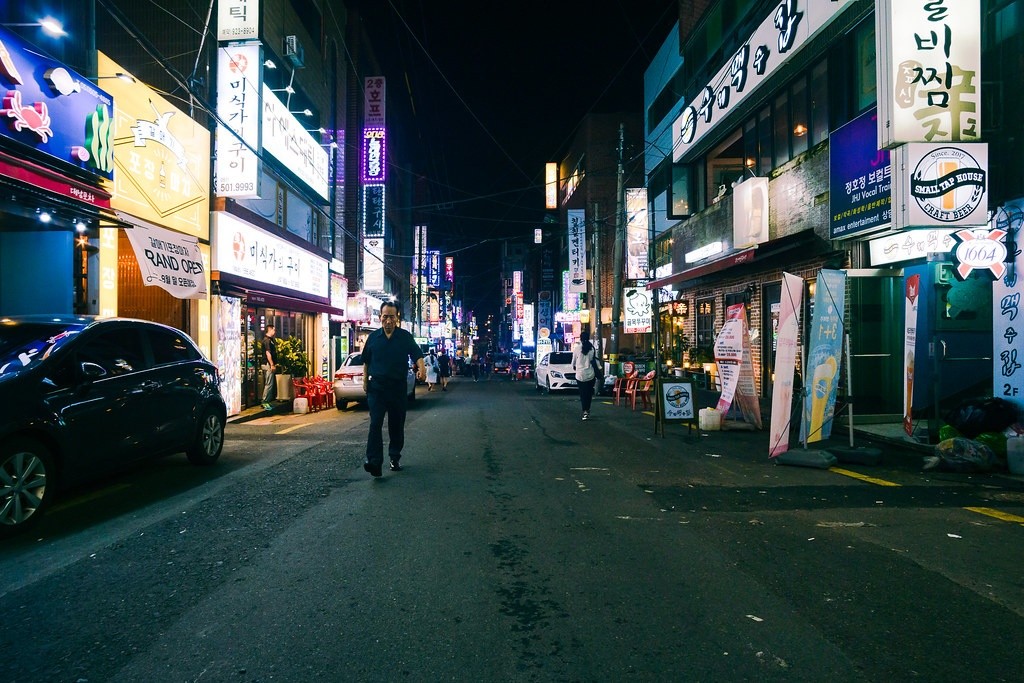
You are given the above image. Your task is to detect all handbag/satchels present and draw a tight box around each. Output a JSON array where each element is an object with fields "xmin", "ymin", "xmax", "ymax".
[
  {"xmin": 446, "ymin": 366, "xmax": 452, "ymax": 375},
  {"xmin": 433, "ymin": 364, "xmax": 440, "ymax": 374},
  {"xmin": 591, "ymin": 346, "xmax": 604, "ymax": 378}
]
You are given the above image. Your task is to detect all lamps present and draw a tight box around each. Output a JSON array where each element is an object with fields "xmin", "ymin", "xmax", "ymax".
[
  {"xmin": 1, "ymin": 16, "xmax": 66, "ymax": 34},
  {"xmin": 86, "ymin": 73, "xmax": 136, "ymax": 84},
  {"xmin": 746, "ymin": 158, "xmax": 755, "ymax": 169},
  {"xmin": 263, "ymin": 59, "xmax": 338, "ymax": 148},
  {"xmin": 793, "ymin": 125, "xmax": 807, "ymax": 136}
]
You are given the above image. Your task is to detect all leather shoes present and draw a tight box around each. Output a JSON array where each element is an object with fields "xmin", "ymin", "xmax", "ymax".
[
  {"xmin": 389, "ymin": 460, "xmax": 401, "ymax": 470},
  {"xmin": 364, "ymin": 461, "xmax": 383, "ymax": 477}
]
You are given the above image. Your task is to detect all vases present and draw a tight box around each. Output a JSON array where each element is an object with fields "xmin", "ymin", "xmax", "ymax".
[
  {"xmin": 275, "ymin": 374, "xmax": 295, "ymax": 402},
  {"xmin": 257, "ymin": 375, "xmax": 264, "ymax": 399}
]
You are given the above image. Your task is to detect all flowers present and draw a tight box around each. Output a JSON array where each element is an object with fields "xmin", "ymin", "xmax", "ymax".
[
  {"xmin": 275, "ymin": 334, "xmax": 311, "ymax": 377},
  {"xmin": 250, "ymin": 339, "xmax": 262, "ymax": 370}
]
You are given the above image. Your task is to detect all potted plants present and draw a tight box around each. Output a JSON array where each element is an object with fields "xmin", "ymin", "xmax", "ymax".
[{"xmin": 674, "ymin": 326, "xmax": 707, "ymax": 388}]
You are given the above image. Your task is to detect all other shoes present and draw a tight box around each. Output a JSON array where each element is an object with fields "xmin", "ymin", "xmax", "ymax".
[
  {"xmin": 431, "ymin": 389, "xmax": 435, "ymax": 391},
  {"xmin": 582, "ymin": 412, "xmax": 590, "ymax": 421},
  {"xmin": 428, "ymin": 385, "xmax": 431, "ymax": 391},
  {"xmin": 441, "ymin": 387, "xmax": 447, "ymax": 391}
]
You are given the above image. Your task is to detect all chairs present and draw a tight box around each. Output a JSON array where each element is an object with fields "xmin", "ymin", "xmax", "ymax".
[
  {"xmin": 613, "ymin": 370, "xmax": 656, "ymax": 411},
  {"xmin": 292, "ymin": 375, "xmax": 334, "ymax": 412}
]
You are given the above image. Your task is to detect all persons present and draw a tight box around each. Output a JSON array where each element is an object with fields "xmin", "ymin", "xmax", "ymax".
[
  {"xmin": 364, "ymin": 302, "xmax": 426, "ymax": 477},
  {"xmin": 570, "ymin": 332, "xmax": 603, "ymax": 421},
  {"xmin": 260, "ymin": 325, "xmax": 277, "ymax": 411},
  {"xmin": 424, "ymin": 347, "xmax": 519, "ymax": 391}
]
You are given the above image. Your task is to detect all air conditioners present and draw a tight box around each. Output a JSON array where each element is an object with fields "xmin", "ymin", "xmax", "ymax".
[{"xmin": 286, "ymin": 35, "xmax": 304, "ymax": 67}]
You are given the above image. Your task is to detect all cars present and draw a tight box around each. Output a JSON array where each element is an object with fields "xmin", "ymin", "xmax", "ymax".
[
  {"xmin": 0, "ymin": 313, "xmax": 227, "ymax": 537},
  {"xmin": 493, "ymin": 355, "xmax": 535, "ymax": 377},
  {"xmin": 333, "ymin": 352, "xmax": 417, "ymax": 409},
  {"xmin": 532, "ymin": 351, "xmax": 578, "ymax": 393}
]
